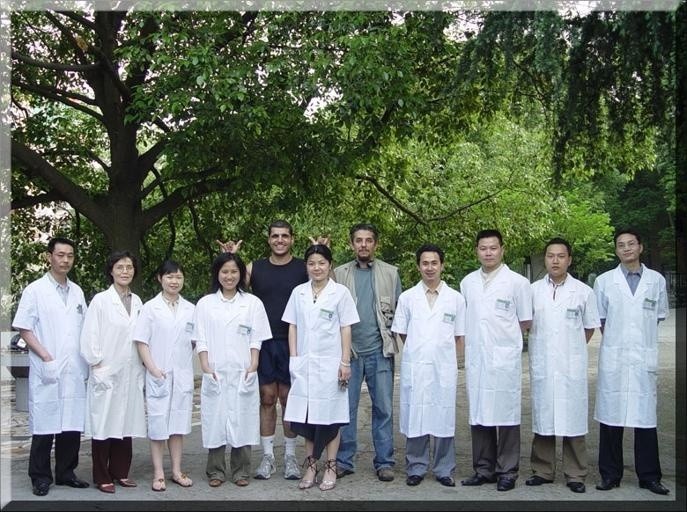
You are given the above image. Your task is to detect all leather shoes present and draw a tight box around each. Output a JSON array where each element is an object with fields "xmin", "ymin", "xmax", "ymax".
[
  {"xmin": 639, "ymin": 480, "xmax": 670, "ymax": 495},
  {"xmin": 568, "ymin": 481, "xmax": 586, "ymax": 493},
  {"xmin": 99, "ymin": 483, "xmax": 115, "ymax": 493},
  {"xmin": 32, "ymin": 480, "xmax": 49, "ymax": 496},
  {"xmin": 119, "ymin": 479, "xmax": 137, "ymax": 487},
  {"xmin": 497, "ymin": 478, "xmax": 515, "ymax": 491},
  {"xmin": 596, "ymin": 479, "xmax": 620, "ymax": 490},
  {"xmin": 461, "ymin": 473, "xmax": 494, "ymax": 485},
  {"xmin": 55, "ymin": 476, "xmax": 88, "ymax": 488},
  {"xmin": 440, "ymin": 476, "xmax": 455, "ymax": 487},
  {"xmin": 525, "ymin": 476, "xmax": 552, "ymax": 486},
  {"xmin": 406, "ymin": 476, "xmax": 421, "ymax": 486}
]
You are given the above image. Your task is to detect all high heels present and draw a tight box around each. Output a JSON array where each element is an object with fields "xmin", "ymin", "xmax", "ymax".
[
  {"xmin": 299, "ymin": 457, "xmax": 319, "ymax": 488},
  {"xmin": 319, "ymin": 459, "xmax": 338, "ymax": 490}
]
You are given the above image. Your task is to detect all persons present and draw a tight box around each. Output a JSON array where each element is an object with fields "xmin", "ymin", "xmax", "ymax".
[
  {"xmin": 593, "ymin": 229, "xmax": 673, "ymax": 494},
  {"xmin": 281, "ymin": 243, "xmax": 362, "ymax": 489},
  {"xmin": 216, "ymin": 221, "xmax": 337, "ymax": 480},
  {"xmin": 13, "ymin": 237, "xmax": 88, "ymax": 495},
  {"xmin": 134, "ymin": 261, "xmax": 195, "ymax": 491},
  {"xmin": 79, "ymin": 251, "xmax": 145, "ymax": 495},
  {"xmin": 390, "ymin": 244, "xmax": 466, "ymax": 487},
  {"xmin": 460, "ymin": 230, "xmax": 532, "ymax": 492},
  {"xmin": 331, "ymin": 225, "xmax": 402, "ymax": 481},
  {"xmin": 193, "ymin": 252, "xmax": 274, "ymax": 486},
  {"xmin": 526, "ymin": 238, "xmax": 601, "ymax": 493}
]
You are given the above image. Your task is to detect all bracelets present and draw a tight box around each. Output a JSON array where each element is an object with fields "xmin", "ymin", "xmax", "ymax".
[{"xmin": 341, "ymin": 360, "xmax": 351, "ymax": 367}]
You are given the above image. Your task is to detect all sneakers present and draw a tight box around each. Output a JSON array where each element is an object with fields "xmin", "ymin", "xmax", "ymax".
[
  {"xmin": 334, "ymin": 464, "xmax": 353, "ymax": 478},
  {"xmin": 254, "ymin": 453, "xmax": 278, "ymax": 478},
  {"xmin": 238, "ymin": 478, "xmax": 249, "ymax": 487},
  {"xmin": 377, "ymin": 466, "xmax": 393, "ymax": 481},
  {"xmin": 210, "ymin": 479, "xmax": 222, "ymax": 487},
  {"xmin": 283, "ymin": 455, "xmax": 301, "ymax": 479}
]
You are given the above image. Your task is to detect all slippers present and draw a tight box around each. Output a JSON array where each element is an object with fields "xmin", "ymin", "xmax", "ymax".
[
  {"xmin": 152, "ymin": 478, "xmax": 167, "ymax": 492},
  {"xmin": 176, "ymin": 473, "xmax": 192, "ymax": 487}
]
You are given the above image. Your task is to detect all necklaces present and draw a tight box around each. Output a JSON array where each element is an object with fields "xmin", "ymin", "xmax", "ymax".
[
  {"xmin": 313, "ymin": 286, "xmax": 323, "ymax": 305},
  {"xmin": 163, "ymin": 294, "xmax": 181, "ymax": 306}
]
